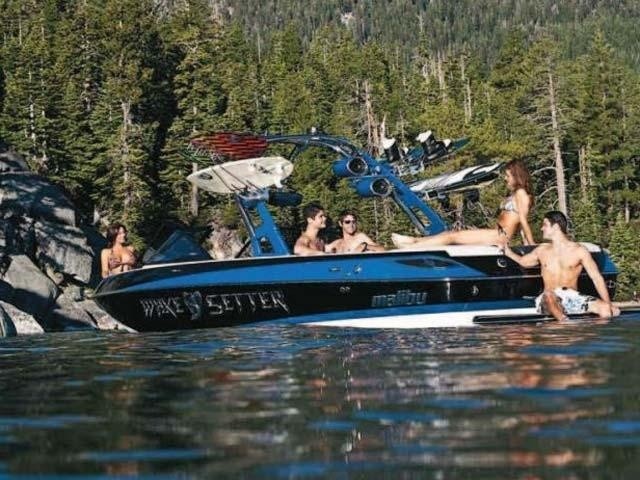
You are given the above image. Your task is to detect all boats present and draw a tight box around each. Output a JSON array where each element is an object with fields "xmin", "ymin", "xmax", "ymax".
[{"xmin": 92, "ymin": 125, "xmax": 621, "ymax": 332}]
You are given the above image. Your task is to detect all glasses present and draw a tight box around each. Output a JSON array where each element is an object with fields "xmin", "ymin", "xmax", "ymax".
[{"xmin": 343, "ymin": 220, "xmax": 356, "ymax": 224}]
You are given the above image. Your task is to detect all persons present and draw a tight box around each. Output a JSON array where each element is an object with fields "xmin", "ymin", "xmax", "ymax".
[
  {"xmin": 293, "ymin": 202, "xmax": 384, "ymax": 256},
  {"xmin": 499, "ymin": 211, "xmax": 621, "ymax": 322},
  {"xmin": 391, "ymin": 158, "xmax": 539, "ymax": 249},
  {"xmin": 100, "ymin": 224, "xmax": 136, "ymax": 279}
]
